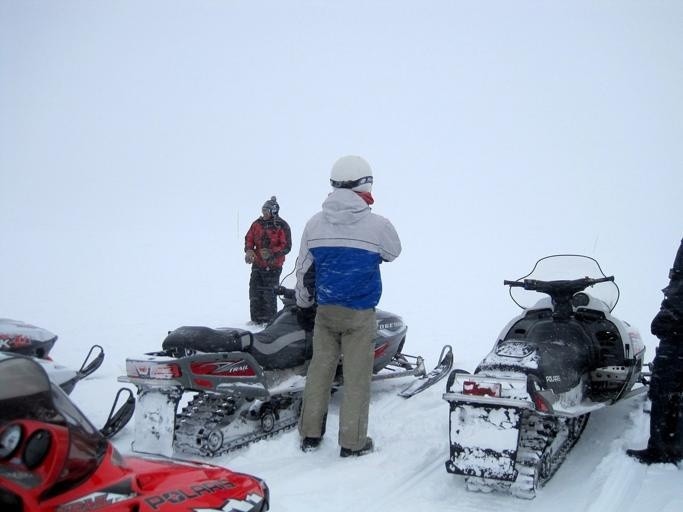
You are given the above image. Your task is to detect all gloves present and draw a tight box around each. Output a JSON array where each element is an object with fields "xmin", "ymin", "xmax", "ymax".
[
  {"xmin": 650, "ymin": 300, "xmax": 677, "ymax": 341},
  {"xmin": 245, "ymin": 250, "xmax": 257, "ymax": 264},
  {"xmin": 260, "ymin": 248, "xmax": 273, "ymax": 262},
  {"xmin": 296, "ymin": 302, "xmax": 318, "ymax": 332}
]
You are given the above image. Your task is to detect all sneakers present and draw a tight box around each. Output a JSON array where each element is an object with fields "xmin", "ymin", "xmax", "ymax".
[
  {"xmin": 626, "ymin": 446, "xmax": 680, "ymax": 466},
  {"xmin": 340, "ymin": 438, "xmax": 372, "ymax": 458},
  {"xmin": 301, "ymin": 436, "xmax": 321, "ymax": 452}
]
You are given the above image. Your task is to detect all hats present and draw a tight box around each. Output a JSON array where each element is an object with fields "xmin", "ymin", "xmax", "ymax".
[{"xmin": 262, "ymin": 197, "xmax": 279, "ymax": 217}]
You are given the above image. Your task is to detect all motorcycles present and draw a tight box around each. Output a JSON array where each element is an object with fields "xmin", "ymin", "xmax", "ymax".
[
  {"xmin": 440, "ymin": 253, "xmax": 652, "ymax": 502},
  {"xmin": 1, "ymin": 356, "xmax": 270, "ymax": 512},
  {"xmin": 0, "ymin": 317, "xmax": 136, "ymax": 441},
  {"xmin": 117, "ymin": 285, "xmax": 452, "ymax": 461}
]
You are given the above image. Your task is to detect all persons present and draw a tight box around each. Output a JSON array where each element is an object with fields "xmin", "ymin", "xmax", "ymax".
[
  {"xmin": 626, "ymin": 237, "xmax": 683, "ymax": 464},
  {"xmin": 294, "ymin": 153, "xmax": 404, "ymax": 457},
  {"xmin": 244, "ymin": 195, "xmax": 293, "ymax": 327}
]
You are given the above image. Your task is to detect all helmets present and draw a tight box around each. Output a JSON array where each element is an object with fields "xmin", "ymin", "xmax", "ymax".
[{"xmin": 330, "ymin": 155, "xmax": 374, "ymax": 193}]
